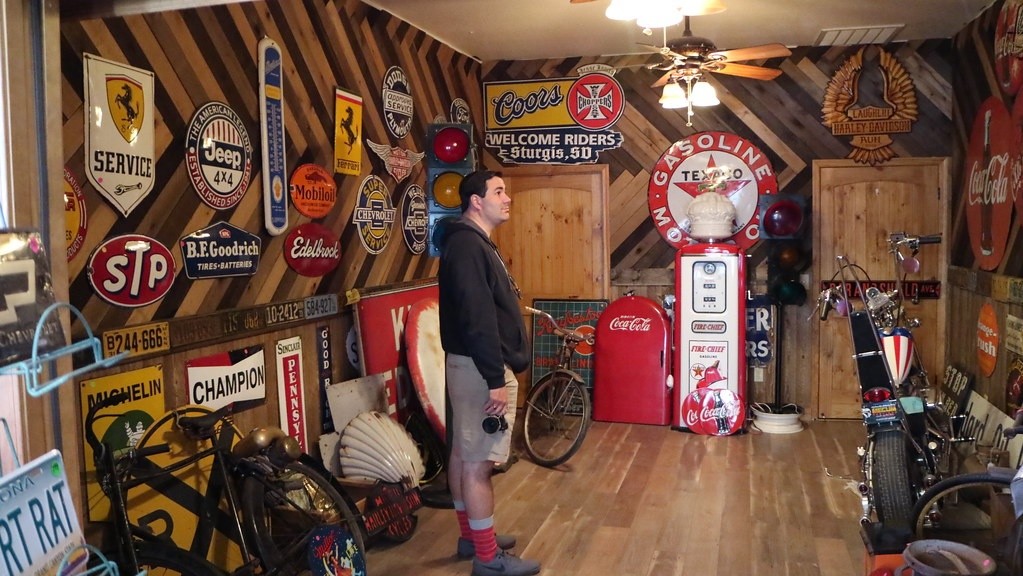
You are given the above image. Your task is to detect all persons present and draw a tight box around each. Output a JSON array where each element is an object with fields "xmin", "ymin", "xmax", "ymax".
[{"xmin": 431, "ymin": 171, "xmax": 541, "ymax": 576}]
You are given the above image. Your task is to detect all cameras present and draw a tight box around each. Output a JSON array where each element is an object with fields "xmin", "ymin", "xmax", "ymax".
[{"xmin": 481, "ymin": 406, "xmax": 508, "ymax": 434}]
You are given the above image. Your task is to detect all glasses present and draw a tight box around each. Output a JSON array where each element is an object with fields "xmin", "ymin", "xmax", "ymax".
[{"xmin": 509, "ymin": 274, "xmax": 522, "ymax": 299}]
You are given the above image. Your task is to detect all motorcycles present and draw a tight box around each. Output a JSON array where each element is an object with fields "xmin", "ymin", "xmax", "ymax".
[{"xmin": 807, "ymin": 232, "xmax": 978, "ymax": 543}]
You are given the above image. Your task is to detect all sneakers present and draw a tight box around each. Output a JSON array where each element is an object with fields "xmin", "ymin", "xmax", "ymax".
[
  {"xmin": 457, "ymin": 532, "xmax": 516, "ymax": 560},
  {"xmin": 471, "ymin": 546, "xmax": 541, "ymax": 576}
]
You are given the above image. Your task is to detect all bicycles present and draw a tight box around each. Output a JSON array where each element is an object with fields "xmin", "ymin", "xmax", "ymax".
[
  {"xmin": 521, "ymin": 306, "xmax": 596, "ymax": 468},
  {"xmin": 909, "ymin": 401, "xmax": 1023, "ymax": 576},
  {"xmin": 83, "ymin": 393, "xmax": 367, "ymax": 576}
]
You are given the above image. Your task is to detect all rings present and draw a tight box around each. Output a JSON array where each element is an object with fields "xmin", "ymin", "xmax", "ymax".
[{"xmin": 493, "ymin": 405, "xmax": 497, "ymax": 409}]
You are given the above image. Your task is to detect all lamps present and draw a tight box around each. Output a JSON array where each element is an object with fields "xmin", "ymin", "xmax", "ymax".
[
  {"xmin": 605, "ymin": 0, "xmax": 727, "ymax": 28},
  {"xmin": 658, "ymin": 72, "xmax": 720, "ymax": 109}
]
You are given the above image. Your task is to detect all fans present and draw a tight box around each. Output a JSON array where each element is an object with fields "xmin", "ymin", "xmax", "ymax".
[{"xmin": 568, "ymin": 0, "xmax": 793, "ymax": 88}]
